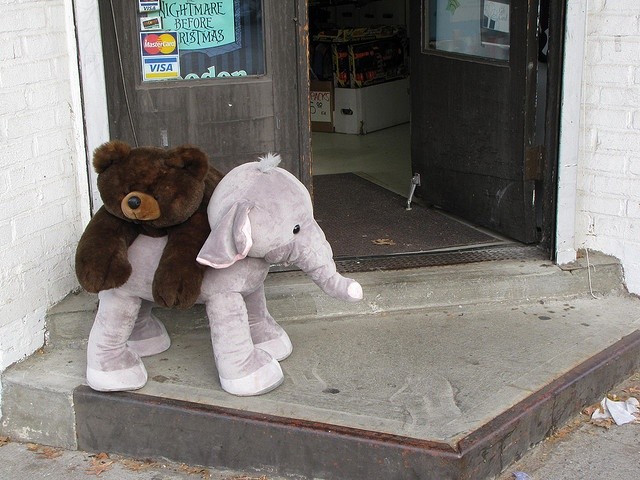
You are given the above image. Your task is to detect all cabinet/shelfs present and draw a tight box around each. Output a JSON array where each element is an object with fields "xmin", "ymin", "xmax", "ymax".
[{"xmin": 311, "ymin": 37, "xmax": 409, "ymax": 135}]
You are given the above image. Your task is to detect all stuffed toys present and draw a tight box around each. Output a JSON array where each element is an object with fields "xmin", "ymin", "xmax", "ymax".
[
  {"xmin": 86, "ymin": 153, "xmax": 363, "ymax": 394},
  {"xmin": 76, "ymin": 142, "xmax": 223, "ymax": 307}
]
